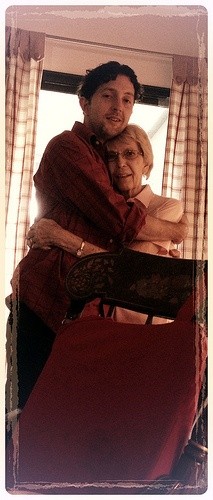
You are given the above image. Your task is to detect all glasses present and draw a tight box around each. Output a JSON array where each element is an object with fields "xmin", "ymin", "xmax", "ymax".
[{"xmin": 108, "ymin": 150, "xmax": 143, "ymax": 162}]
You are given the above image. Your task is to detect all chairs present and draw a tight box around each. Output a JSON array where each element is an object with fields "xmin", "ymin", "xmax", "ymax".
[{"xmin": 15, "ymin": 248, "xmax": 208, "ymax": 492}]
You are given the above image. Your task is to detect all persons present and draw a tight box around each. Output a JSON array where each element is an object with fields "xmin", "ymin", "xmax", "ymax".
[
  {"xmin": 26, "ymin": 123, "xmax": 184, "ymax": 325},
  {"xmin": 2, "ymin": 61, "xmax": 190, "ymax": 439}
]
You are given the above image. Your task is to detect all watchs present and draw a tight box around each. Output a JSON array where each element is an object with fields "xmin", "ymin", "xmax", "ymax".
[{"xmin": 73, "ymin": 239, "xmax": 87, "ymax": 259}]
